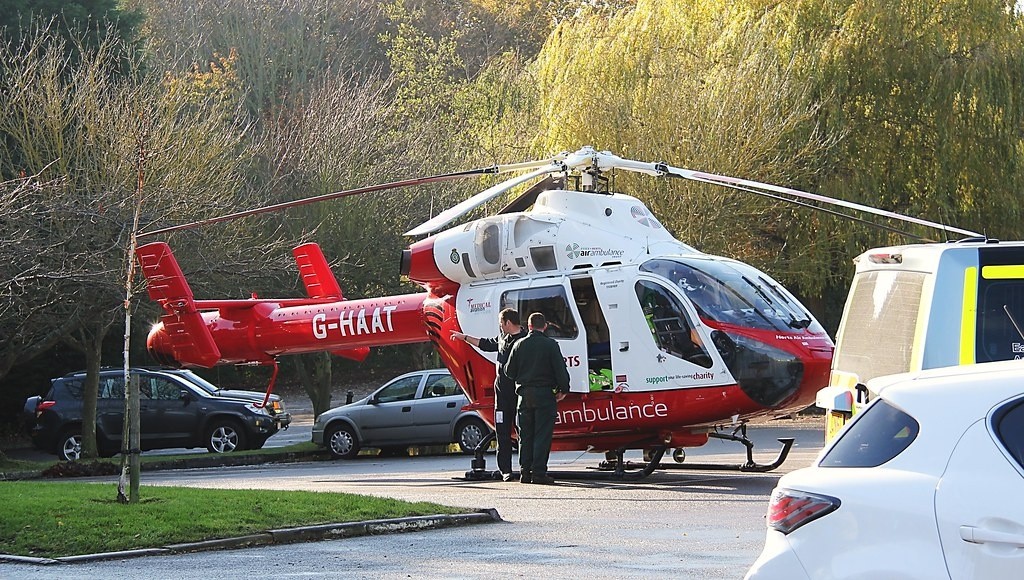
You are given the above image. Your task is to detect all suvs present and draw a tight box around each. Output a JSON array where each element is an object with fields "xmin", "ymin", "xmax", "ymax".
[
  {"xmin": 24, "ymin": 366, "xmax": 294, "ymax": 465},
  {"xmin": 745, "ymin": 357, "xmax": 1024, "ymax": 580}
]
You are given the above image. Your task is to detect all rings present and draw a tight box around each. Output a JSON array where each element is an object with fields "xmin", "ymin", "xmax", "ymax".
[{"xmin": 452, "ymin": 333, "xmax": 454, "ymax": 338}]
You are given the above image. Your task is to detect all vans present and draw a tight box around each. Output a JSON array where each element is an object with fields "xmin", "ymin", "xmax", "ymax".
[{"xmin": 815, "ymin": 239, "xmax": 1024, "ymax": 454}]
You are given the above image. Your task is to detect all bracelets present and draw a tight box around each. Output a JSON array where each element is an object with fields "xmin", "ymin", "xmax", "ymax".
[{"xmin": 464, "ymin": 334, "xmax": 469, "ymax": 341}]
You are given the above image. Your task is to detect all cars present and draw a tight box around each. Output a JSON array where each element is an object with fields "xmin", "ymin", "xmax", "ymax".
[{"xmin": 311, "ymin": 366, "xmax": 494, "ymax": 460}]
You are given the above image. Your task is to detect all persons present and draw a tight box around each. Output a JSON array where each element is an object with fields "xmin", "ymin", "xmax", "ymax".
[
  {"xmin": 503, "ymin": 313, "xmax": 570, "ymax": 484},
  {"xmin": 450, "ymin": 308, "xmax": 528, "ymax": 482},
  {"xmin": 643, "ymin": 280, "xmax": 728, "ymax": 350}
]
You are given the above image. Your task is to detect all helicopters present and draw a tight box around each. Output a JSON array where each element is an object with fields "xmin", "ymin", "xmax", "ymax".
[{"xmin": 133, "ymin": 144, "xmax": 985, "ymax": 481}]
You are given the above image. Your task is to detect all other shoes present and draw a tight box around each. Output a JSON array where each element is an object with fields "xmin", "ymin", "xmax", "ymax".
[
  {"xmin": 502, "ymin": 472, "xmax": 514, "ymax": 481},
  {"xmin": 532, "ymin": 474, "xmax": 554, "ymax": 485},
  {"xmin": 520, "ymin": 472, "xmax": 535, "ymax": 483}
]
